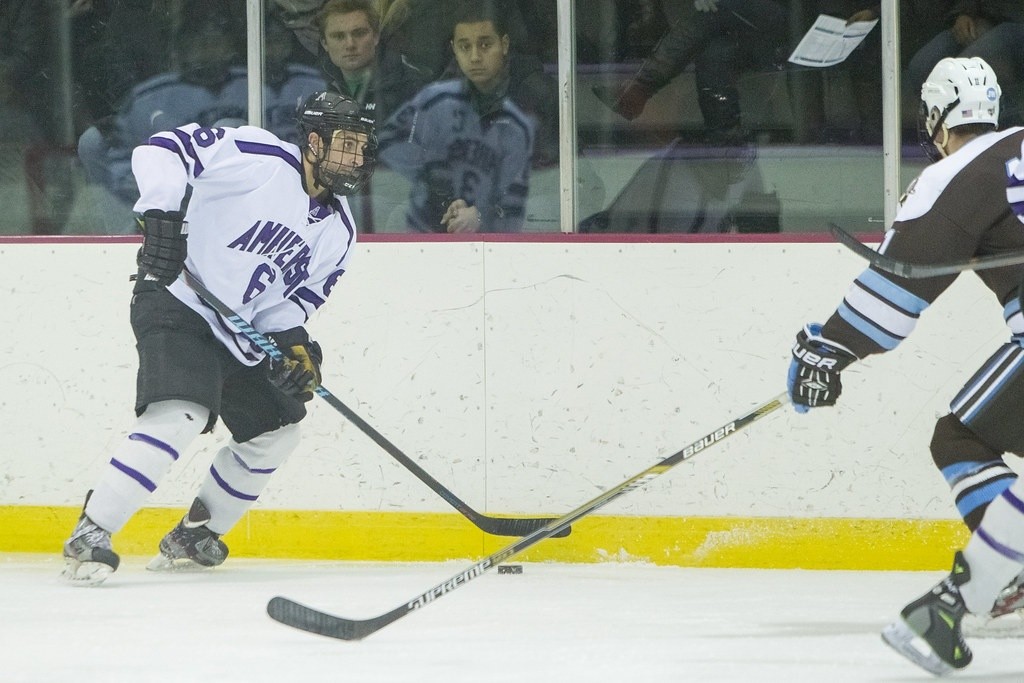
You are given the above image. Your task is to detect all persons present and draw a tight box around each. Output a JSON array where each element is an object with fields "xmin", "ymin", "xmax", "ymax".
[
  {"xmin": 787, "ymin": 56, "xmax": 1024, "ymax": 674},
  {"xmin": 61, "ymin": 90, "xmax": 379, "ymax": 587},
  {"xmin": 317, "ymin": 0, "xmax": 560, "ymax": 172},
  {"xmin": 76, "ymin": 16, "xmax": 532, "ymax": 232},
  {"xmin": 578, "ymin": 88, "xmax": 781, "ymax": 233},
  {"xmin": 592, "ymin": 1, "xmax": 1024, "ymax": 145}
]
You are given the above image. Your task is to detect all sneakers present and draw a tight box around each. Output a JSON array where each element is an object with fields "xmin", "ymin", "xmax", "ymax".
[
  {"xmin": 60, "ymin": 489, "xmax": 120, "ymax": 588},
  {"xmin": 146, "ymin": 497, "xmax": 229, "ymax": 574},
  {"xmin": 881, "ymin": 550, "xmax": 973, "ymax": 676},
  {"xmin": 989, "ymin": 571, "xmax": 1024, "ymax": 620}
]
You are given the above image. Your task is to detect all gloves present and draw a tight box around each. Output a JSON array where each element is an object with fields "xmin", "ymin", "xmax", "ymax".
[
  {"xmin": 740, "ymin": 183, "xmax": 782, "ymax": 232},
  {"xmin": 786, "ymin": 321, "xmax": 861, "ymax": 415},
  {"xmin": 263, "ymin": 325, "xmax": 323, "ymax": 403},
  {"xmin": 134, "ymin": 208, "xmax": 189, "ymax": 287}
]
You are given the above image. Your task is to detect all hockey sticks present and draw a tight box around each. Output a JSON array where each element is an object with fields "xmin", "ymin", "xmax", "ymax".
[
  {"xmin": 177, "ymin": 269, "xmax": 571, "ymax": 540},
  {"xmin": 266, "ymin": 386, "xmax": 796, "ymax": 642},
  {"xmin": 825, "ymin": 220, "xmax": 1024, "ymax": 279}
]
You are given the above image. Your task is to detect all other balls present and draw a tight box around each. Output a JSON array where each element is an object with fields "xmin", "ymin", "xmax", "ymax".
[{"xmin": 498, "ymin": 564, "xmax": 524, "ymax": 575}]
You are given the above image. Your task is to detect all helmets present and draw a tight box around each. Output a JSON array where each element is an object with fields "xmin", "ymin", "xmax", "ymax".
[
  {"xmin": 297, "ymin": 91, "xmax": 375, "ymax": 133},
  {"xmin": 674, "ymin": 88, "xmax": 758, "ymax": 199},
  {"xmin": 176, "ymin": 12, "xmax": 237, "ymax": 86},
  {"xmin": 915, "ymin": 56, "xmax": 1002, "ymax": 163}
]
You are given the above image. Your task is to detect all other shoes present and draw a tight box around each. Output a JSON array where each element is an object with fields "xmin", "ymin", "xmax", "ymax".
[{"xmin": 591, "ymin": 73, "xmax": 654, "ymax": 121}]
